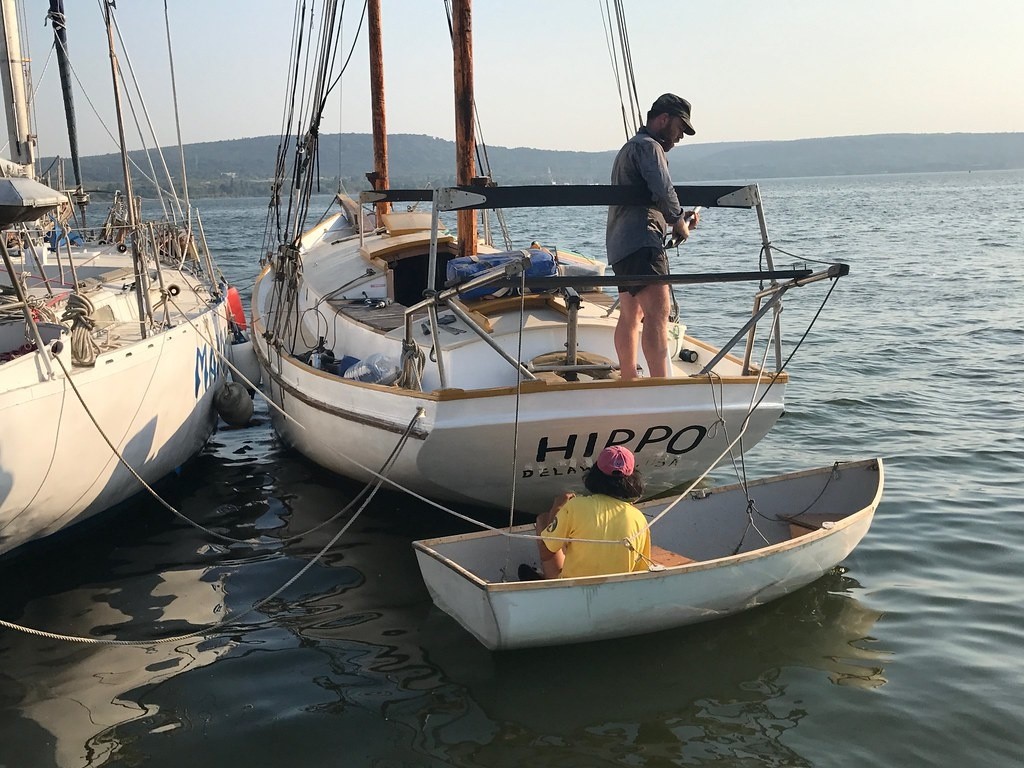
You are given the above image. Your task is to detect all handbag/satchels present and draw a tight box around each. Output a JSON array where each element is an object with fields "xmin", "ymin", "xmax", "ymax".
[{"xmin": 531, "ymin": 241, "xmax": 606, "ymax": 292}]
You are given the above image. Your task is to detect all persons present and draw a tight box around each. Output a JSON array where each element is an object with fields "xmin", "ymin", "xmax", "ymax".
[
  {"xmin": 516, "ymin": 444, "xmax": 660, "ymax": 583},
  {"xmin": 601, "ymin": 90, "xmax": 704, "ymax": 379}
]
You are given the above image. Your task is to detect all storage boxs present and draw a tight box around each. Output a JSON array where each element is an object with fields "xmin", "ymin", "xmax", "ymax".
[{"xmin": 662, "ymin": 322, "xmax": 687, "ymax": 361}]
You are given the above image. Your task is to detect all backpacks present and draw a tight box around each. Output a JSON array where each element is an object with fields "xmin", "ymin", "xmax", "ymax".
[{"xmin": 449, "ymin": 248, "xmax": 559, "ymax": 300}]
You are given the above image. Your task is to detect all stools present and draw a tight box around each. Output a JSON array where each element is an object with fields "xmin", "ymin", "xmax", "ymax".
[
  {"xmin": 652, "ymin": 543, "xmax": 698, "ymax": 569},
  {"xmin": 778, "ymin": 512, "xmax": 852, "ymax": 531}
]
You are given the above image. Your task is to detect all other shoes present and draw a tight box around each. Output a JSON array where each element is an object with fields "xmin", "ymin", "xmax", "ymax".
[{"xmin": 517, "ymin": 564, "xmax": 549, "ymax": 581}]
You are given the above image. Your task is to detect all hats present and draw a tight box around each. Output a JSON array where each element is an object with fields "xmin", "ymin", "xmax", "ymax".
[
  {"xmin": 652, "ymin": 95, "xmax": 696, "ymax": 135},
  {"xmin": 597, "ymin": 446, "xmax": 635, "ymax": 476}
]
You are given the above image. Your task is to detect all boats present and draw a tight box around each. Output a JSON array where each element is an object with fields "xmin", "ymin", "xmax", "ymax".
[
  {"xmin": 2, "ymin": 1, "xmax": 263, "ymax": 557},
  {"xmin": 412, "ymin": 455, "xmax": 885, "ymax": 650},
  {"xmin": 248, "ymin": 2, "xmax": 850, "ymax": 507}
]
